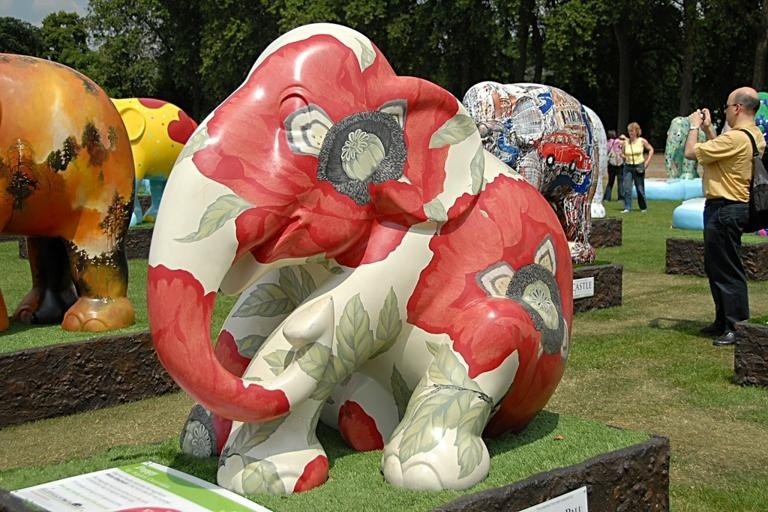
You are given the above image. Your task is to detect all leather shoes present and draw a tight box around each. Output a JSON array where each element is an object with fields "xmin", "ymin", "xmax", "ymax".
[{"xmin": 699, "ymin": 323, "xmax": 736, "ymax": 346}]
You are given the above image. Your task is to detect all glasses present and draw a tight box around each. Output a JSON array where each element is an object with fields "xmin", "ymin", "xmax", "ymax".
[{"xmin": 723, "ymin": 104, "xmax": 743, "ymax": 109}]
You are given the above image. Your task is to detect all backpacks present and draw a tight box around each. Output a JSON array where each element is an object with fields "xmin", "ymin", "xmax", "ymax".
[{"xmin": 731, "ymin": 128, "xmax": 768, "ymax": 233}]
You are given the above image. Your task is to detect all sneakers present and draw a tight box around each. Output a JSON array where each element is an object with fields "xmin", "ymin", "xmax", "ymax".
[
  {"xmin": 604, "ymin": 199, "xmax": 624, "ymax": 202},
  {"xmin": 620, "ymin": 209, "xmax": 648, "ymax": 213}
]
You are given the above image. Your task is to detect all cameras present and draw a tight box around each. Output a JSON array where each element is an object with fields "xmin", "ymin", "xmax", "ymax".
[{"xmin": 701, "ymin": 112, "xmax": 705, "ymax": 120}]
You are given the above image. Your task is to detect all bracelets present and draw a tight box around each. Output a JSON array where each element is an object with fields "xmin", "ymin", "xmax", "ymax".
[{"xmin": 689, "ymin": 127, "xmax": 700, "ymax": 131}]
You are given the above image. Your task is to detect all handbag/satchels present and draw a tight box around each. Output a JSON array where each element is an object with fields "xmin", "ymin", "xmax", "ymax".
[
  {"xmin": 635, "ymin": 167, "xmax": 645, "ymax": 176},
  {"xmin": 608, "ymin": 138, "xmax": 624, "ymax": 167}
]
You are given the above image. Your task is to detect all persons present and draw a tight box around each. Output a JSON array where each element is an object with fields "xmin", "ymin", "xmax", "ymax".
[
  {"xmin": 683, "ymin": 87, "xmax": 767, "ymax": 347},
  {"xmin": 604, "ymin": 129, "xmax": 629, "ymax": 204},
  {"xmin": 620, "ymin": 122, "xmax": 654, "ymax": 214}
]
